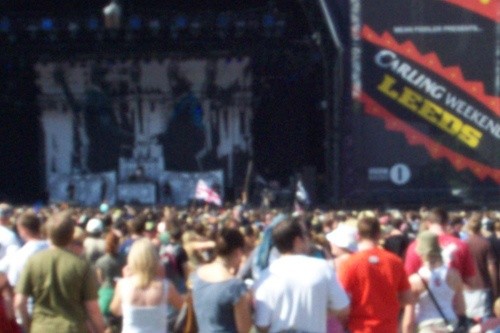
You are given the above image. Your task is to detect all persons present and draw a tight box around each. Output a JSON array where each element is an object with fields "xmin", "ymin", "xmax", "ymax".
[
  {"xmin": 109, "ymin": 238, "xmax": 189, "ymax": 333},
  {"xmin": 2, "ymin": 213, "xmax": 52, "ymax": 320},
  {"xmin": 12, "ymin": 212, "xmax": 112, "ymax": 332},
  {"xmin": 2, "ymin": 205, "xmax": 500, "ymax": 333},
  {"xmin": 337, "ymin": 217, "xmax": 418, "ymax": 333},
  {"xmin": 400, "ymin": 229, "xmax": 466, "ymax": 333},
  {"xmin": 253, "ymin": 219, "xmax": 351, "ymax": 333},
  {"xmin": 185, "ymin": 228, "xmax": 253, "ymax": 333}
]
